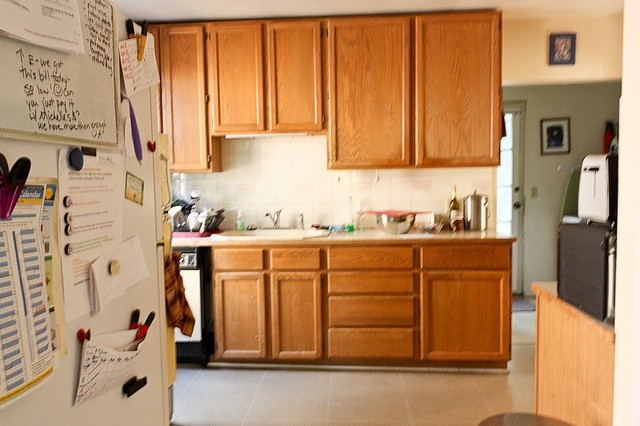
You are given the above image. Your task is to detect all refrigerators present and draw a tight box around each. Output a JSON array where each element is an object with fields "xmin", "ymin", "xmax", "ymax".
[{"xmin": 1, "ymin": 0, "xmax": 174, "ymax": 425}]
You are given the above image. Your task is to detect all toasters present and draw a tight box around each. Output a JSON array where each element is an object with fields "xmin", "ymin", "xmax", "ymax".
[{"xmin": 577, "ymin": 153, "xmax": 617, "ymax": 225}]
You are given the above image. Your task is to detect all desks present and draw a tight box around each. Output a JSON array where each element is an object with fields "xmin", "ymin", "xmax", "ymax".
[{"xmin": 531, "ymin": 281, "xmax": 614, "ymax": 426}]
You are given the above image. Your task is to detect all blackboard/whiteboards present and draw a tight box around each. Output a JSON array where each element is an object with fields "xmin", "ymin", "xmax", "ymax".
[{"xmin": 0, "ymin": 0, "xmax": 121, "ymax": 149}]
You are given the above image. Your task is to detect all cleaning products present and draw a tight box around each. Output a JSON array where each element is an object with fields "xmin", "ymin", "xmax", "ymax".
[{"xmin": 235, "ymin": 208, "xmax": 244, "ymax": 231}]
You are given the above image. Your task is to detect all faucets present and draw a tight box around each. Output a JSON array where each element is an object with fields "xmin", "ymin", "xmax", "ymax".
[
  {"xmin": 264, "ymin": 209, "xmax": 282, "ymax": 228},
  {"xmin": 299, "ymin": 213, "xmax": 304, "ymax": 230}
]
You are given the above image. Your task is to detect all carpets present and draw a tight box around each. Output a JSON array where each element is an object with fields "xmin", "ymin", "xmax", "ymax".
[{"xmin": 511, "ymin": 298, "xmax": 535, "ymax": 313}]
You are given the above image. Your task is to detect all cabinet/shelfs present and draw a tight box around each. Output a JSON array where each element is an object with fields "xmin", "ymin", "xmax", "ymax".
[
  {"xmin": 206, "ymin": 17, "xmax": 325, "ymax": 137},
  {"xmin": 141, "ymin": 21, "xmax": 222, "ymax": 174},
  {"xmin": 325, "ymin": 8, "xmax": 502, "ymax": 171},
  {"xmin": 420, "ymin": 238, "xmax": 513, "ymax": 367},
  {"xmin": 208, "ymin": 244, "xmax": 324, "ymax": 364},
  {"xmin": 322, "ymin": 240, "xmax": 420, "ymax": 366}
]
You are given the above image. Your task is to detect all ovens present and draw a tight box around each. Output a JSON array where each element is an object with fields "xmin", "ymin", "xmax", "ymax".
[{"xmin": 174, "ymin": 246, "xmax": 212, "ymax": 367}]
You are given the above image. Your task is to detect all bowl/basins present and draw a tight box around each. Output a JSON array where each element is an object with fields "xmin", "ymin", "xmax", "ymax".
[{"xmin": 381, "ymin": 213, "xmax": 416, "ymax": 234}]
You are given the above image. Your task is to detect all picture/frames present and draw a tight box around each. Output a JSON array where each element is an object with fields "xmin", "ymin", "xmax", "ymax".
[
  {"xmin": 549, "ymin": 33, "xmax": 575, "ymax": 65},
  {"xmin": 541, "ymin": 117, "xmax": 571, "ymax": 155}
]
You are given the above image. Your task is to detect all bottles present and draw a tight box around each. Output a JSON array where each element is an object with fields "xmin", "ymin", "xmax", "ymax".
[{"xmin": 448, "ymin": 184, "xmax": 460, "ymax": 231}]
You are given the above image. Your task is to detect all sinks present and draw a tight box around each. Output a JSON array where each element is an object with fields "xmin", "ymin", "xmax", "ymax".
[{"xmin": 213, "ymin": 227, "xmax": 331, "ymax": 240}]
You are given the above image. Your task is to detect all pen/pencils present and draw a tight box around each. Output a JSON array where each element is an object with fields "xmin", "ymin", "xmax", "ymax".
[{"xmin": 124, "ymin": 97, "xmax": 143, "ymax": 166}]
